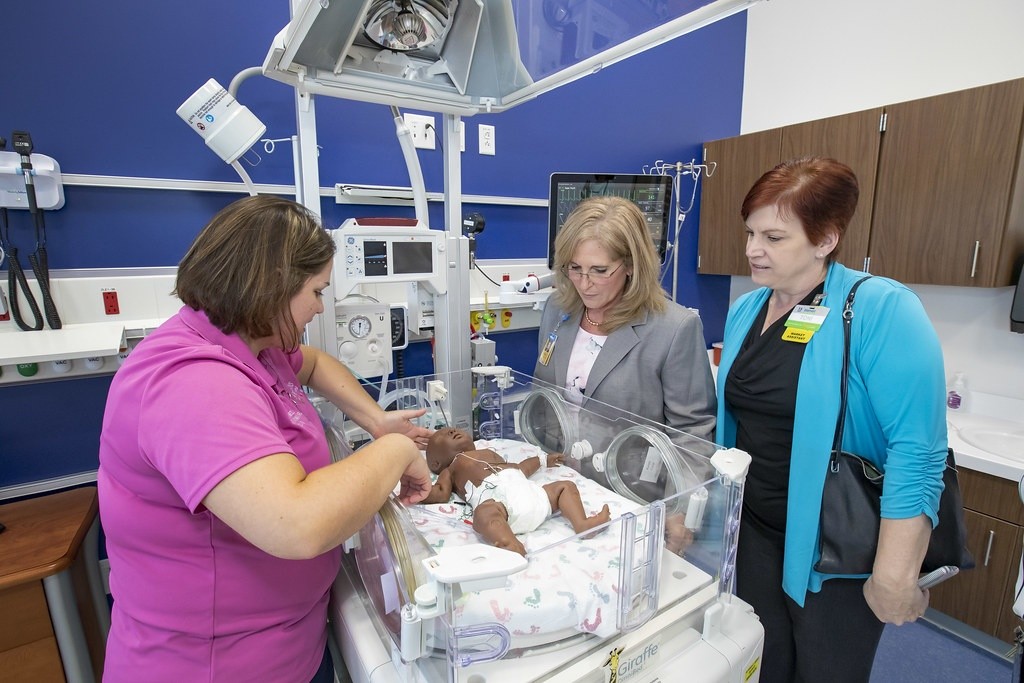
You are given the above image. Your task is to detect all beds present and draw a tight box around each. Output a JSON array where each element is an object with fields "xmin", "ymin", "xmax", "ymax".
[{"xmin": 303, "ymin": 366, "xmax": 764, "ymax": 683}]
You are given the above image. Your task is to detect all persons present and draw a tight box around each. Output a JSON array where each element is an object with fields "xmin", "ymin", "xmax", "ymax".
[
  {"xmin": 421, "ymin": 426, "xmax": 612, "ymax": 557},
  {"xmin": 96, "ymin": 195, "xmax": 433, "ymax": 683},
  {"xmin": 529, "ymin": 196, "xmax": 718, "ymax": 553},
  {"xmin": 714, "ymin": 156, "xmax": 949, "ymax": 683}
]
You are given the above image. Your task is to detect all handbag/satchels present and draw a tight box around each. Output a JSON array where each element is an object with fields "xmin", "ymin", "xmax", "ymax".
[{"xmin": 813, "ymin": 449, "xmax": 975, "ymax": 574}]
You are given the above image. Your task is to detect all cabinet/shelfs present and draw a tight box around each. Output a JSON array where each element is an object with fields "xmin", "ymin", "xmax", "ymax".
[
  {"xmin": 697, "ymin": 76, "xmax": 1024, "ymax": 289},
  {"xmin": 917, "ymin": 466, "xmax": 1024, "ymax": 645}
]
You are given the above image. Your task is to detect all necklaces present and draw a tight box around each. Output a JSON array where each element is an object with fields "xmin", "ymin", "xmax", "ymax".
[{"xmin": 585, "ymin": 307, "xmax": 602, "ymax": 326}]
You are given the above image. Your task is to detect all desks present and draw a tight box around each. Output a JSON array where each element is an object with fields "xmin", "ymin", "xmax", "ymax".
[{"xmin": 0, "ymin": 486, "xmax": 111, "ymax": 683}]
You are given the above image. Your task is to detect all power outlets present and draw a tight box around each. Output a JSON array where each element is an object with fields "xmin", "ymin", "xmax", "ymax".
[{"xmin": 478, "ymin": 124, "xmax": 496, "ymax": 156}]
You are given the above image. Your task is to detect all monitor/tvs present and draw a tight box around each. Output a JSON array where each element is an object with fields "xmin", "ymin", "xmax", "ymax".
[{"xmin": 547, "ymin": 171, "xmax": 673, "ymax": 270}]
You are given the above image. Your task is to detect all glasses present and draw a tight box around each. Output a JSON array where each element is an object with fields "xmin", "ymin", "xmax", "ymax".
[{"xmin": 560, "ymin": 255, "xmax": 626, "ymax": 285}]
